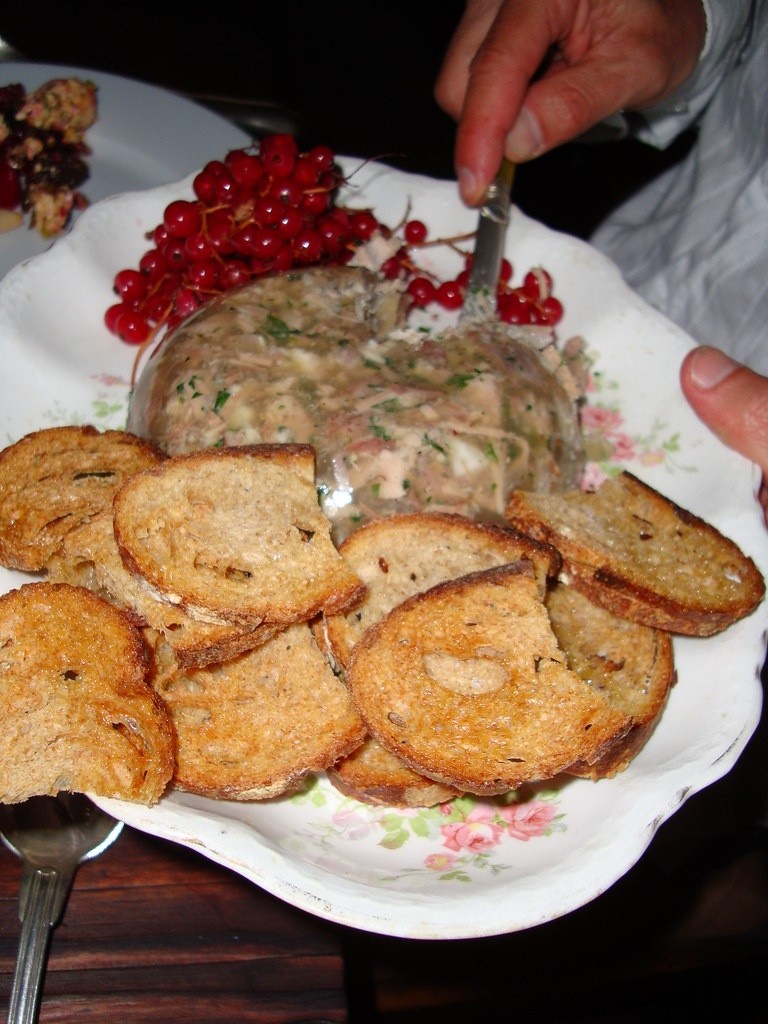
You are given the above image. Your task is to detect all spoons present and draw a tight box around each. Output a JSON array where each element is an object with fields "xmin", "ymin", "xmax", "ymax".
[{"xmin": 1, "ymin": 792, "xmax": 124, "ymax": 1024}]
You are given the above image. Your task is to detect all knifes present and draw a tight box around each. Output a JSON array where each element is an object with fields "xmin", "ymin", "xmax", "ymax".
[{"xmin": 455, "ymin": 154, "xmax": 516, "ymax": 331}]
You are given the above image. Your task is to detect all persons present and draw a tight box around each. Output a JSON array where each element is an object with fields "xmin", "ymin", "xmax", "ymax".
[{"xmin": 435, "ymin": 0, "xmax": 768, "ymax": 534}]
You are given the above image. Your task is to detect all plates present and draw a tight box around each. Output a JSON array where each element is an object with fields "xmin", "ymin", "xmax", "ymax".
[
  {"xmin": 1, "ymin": 150, "xmax": 766, "ymax": 942},
  {"xmin": 1, "ymin": 61, "xmax": 255, "ymax": 286}
]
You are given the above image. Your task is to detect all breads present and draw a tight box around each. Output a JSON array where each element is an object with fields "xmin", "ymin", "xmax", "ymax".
[{"xmin": 1, "ymin": 424, "xmax": 765, "ymax": 806}]
